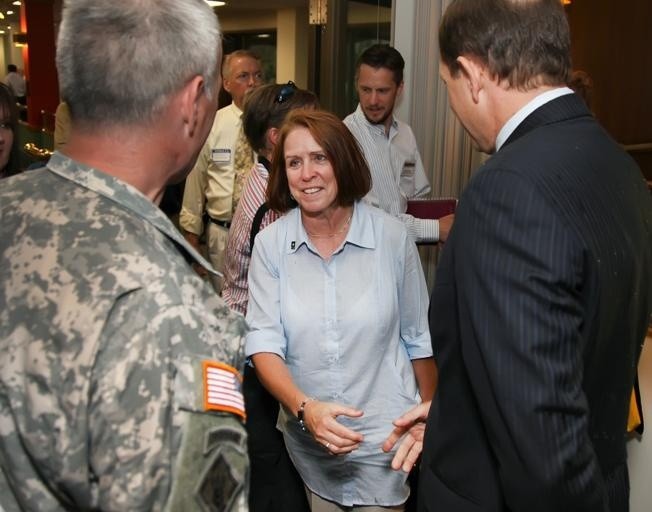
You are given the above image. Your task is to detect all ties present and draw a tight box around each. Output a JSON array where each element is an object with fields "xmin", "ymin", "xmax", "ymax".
[{"xmin": 233, "ymin": 115, "xmax": 253, "ymax": 214}]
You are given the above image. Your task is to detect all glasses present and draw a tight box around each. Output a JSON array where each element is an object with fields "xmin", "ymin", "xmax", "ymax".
[{"xmin": 276, "ymin": 81, "xmax": 297, "ymax": 103}]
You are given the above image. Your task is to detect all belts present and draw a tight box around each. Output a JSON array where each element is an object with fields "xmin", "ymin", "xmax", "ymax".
[{"xmin": 210, "ymin": 218, "xmax": 231, "ymax": 229}]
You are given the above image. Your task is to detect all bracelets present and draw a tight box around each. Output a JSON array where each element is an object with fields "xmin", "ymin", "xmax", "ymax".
[{"xmin": 296, "ymin": 394, "xmax": 316, "ymax": 438}]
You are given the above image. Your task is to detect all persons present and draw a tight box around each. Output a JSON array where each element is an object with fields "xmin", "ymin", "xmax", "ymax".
[
  {"xmin": 219, "ymin": 80, "xmax": 320, "ymax": 511},
  {"xmin": 561, "ymin": 68, "xmax": 646, "ymax": 444},
  {"xmin": 421, "ymin": 0, "xmax": 650, "ymax": 510},
  {"xmin": 1, "ymin": 82, "xmax": 28, "ymax": 178},
  {"xmin": 1, "ymin": 0, "xmax": 251, "ymax": 512},
  {"xmin": 243, "ymin": 110, "xmax": 441, "ymax": 511},
  {"xmin": 175, "ymin": 50, "xmax": 266, "ymax": 299},
  {"xmin": 2, "ymin": 64, "xmax": 28, "ymax": 124},
  {"xmin": 339, "ymin": 43, "xmax": 457, "ymax": 248}
]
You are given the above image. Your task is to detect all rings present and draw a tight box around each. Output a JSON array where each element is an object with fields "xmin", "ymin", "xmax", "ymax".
[{"xmin": 323, "ymin": 440, "xmax": 331, "ymax": 449}]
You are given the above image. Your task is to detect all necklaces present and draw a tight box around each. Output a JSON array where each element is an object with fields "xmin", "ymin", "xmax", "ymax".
[{"xmin": 302, "ymin": 214, "xmax": 353, "ymax": 239}]
